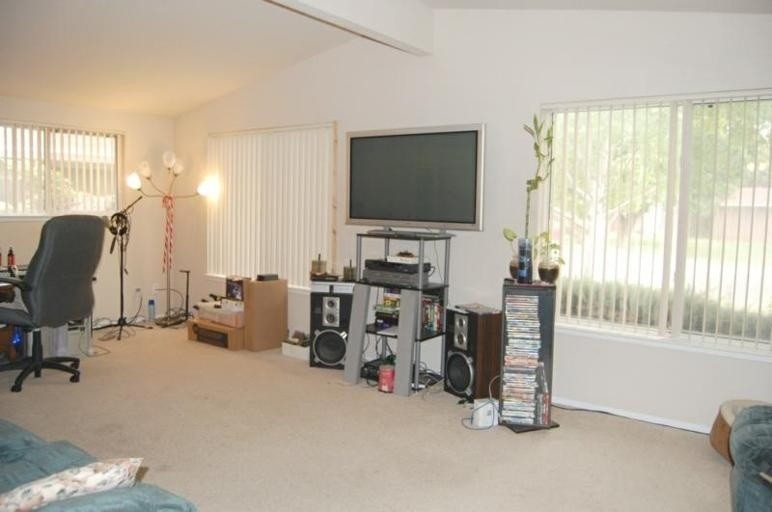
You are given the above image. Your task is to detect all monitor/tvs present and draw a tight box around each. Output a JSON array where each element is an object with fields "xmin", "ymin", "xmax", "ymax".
[{"xmin": 344, "ymin": 123, "xmax": 485, "ymax": 238}]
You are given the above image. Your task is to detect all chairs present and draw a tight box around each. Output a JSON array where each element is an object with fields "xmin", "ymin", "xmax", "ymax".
[
  {"xmin": 0, "ymin": 214, "xmax": 106, "ymax": 392},
  {"xmin": 729, "ymin": 404, "xmax": 772, "ymax": 512}
]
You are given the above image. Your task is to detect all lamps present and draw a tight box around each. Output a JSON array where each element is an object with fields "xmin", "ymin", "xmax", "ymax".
[{"xmin": 125, "ymin": 151, "xmax": 218, "ymax": 328}]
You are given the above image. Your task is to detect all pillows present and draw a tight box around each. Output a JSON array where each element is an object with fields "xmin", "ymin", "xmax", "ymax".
[{"xmin": 0, "ymin": 457, "xmax": 144, "ymax": 512}]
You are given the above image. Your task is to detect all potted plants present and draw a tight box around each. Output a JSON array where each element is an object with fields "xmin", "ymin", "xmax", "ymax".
[
  {"xmin": 519, "ymin": 114, "xmax": 556, "ymax": 282},
  {"xmin": 533, "ymin": 231, "xmax": 565, "ymax": 284},
  {"xmin": 502, "ymin": 226, "xmax": 519, "ymax": 282}
]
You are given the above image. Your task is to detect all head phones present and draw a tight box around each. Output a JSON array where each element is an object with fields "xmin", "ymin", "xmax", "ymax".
[{"xmin": 109, "ymin": 213, "xmax": 127, "ymax": 235}]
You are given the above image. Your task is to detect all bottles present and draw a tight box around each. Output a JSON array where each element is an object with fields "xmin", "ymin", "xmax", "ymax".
[
  {"xmin": 147, "ymin": 299, "xmax": 155, "ymax": 322},
  {"xmin": 377, "ymin": 366, "xmax": 394, "ymax": 392},
  {"xmin": 7, "ymin": 246, "xmax": 15, "ymax": 265},
  {"xmin": 517, "ymin": 238, "xmax": 533, "ymax": 284}
]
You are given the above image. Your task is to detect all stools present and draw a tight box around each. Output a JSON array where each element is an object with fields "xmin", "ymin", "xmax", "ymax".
[{"xmin": 709, "ymin": 400, "xmax": 772, "ymax": 461}]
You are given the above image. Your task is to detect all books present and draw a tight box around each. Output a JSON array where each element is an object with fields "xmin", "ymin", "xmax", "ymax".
[
  {"xmin": 372, "ymin": 291, "xmax": 441, "ymax": 332},
  {"xmin": 500, "ymin": 294, "xmax": 550, "ymax": 428}
]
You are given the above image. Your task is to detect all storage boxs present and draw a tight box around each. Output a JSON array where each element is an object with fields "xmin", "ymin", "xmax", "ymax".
[{"xmin": 196, "ymin": 298, "xmax": 244, "ymax": 328}]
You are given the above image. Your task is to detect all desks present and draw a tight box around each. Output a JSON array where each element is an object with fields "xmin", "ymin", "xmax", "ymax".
[{"xmin": 185, "ymin": 318, "xmax": 245, "ymax": 351}]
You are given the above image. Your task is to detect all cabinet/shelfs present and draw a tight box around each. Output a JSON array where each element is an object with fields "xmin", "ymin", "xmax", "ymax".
[
  {"xmin": 243, "ymin": 277, "xmax": 289, "ymax": 350},
  {"xmin": 355, "ymin": 231, "xmax": 455, "ymax": 394}
]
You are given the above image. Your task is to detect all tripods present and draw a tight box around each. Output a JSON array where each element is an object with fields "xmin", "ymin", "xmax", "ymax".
[{"xmin": 91, "ymin": 196, "xmax": 153, "ymax": 340}]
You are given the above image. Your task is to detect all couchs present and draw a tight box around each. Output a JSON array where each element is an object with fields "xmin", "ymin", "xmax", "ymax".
[{"xmin": 0, "ymin": 419, "xmax": 195, "ymax": 512}]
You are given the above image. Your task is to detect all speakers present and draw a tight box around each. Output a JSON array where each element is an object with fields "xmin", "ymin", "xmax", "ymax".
[
  {"xmin": 310, "ymin": 284, "xmax": 353, "ymax": 370},
  {"xmin": 443, "ymin": 302, "xmax": 503, "ymax": 401}
]
